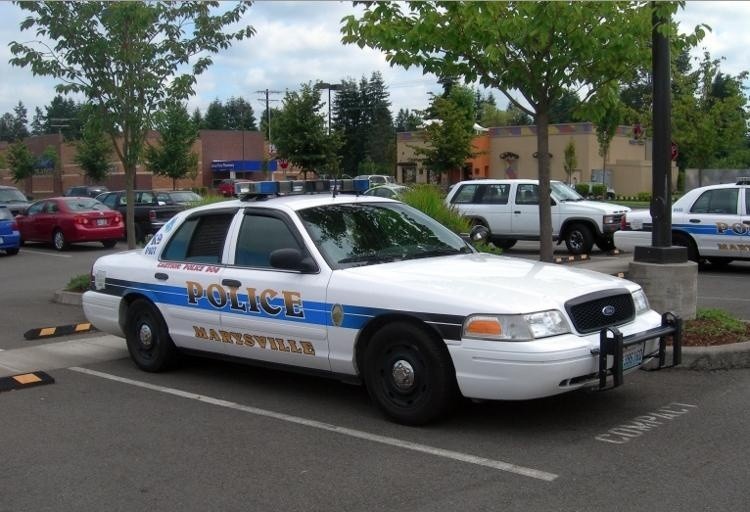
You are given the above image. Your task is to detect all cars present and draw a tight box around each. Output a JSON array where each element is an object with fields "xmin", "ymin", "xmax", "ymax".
[
  {"xmin": 613, "ymin": 179, "xmax": 750, "ymax": 278},
  {"xmin": 0, "ymin": 182, "xmax": 210, "ymax": 259},
  {"xmin": 570, "ymin": 180, "xmax": 619, "ymax": 204},
  {"xmin": 218, "ymin": 173, "xmax": 412, "ymax": 202},
  {"xmin": 77, "ymin": 176, "xmax": 688, "ymax": 428}
]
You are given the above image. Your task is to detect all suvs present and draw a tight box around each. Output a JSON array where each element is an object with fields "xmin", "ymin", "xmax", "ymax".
[{"xmin": 440, "ymin": 177, "xmax": 635, "ymax": 258}]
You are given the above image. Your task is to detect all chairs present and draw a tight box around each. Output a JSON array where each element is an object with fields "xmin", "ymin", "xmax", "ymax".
[
  {"xmin": 186, "ymin": 216, "xmax": 233, "ymax": 264},
  {"xmin": 482, "ymin": 187, "xmax": 533, "ymax": 199}
]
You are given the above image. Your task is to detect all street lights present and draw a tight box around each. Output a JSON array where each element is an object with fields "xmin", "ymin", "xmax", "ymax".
[{"xmin": 315, "ymin": 82, "xmax": 345, "ymax": 137}]
